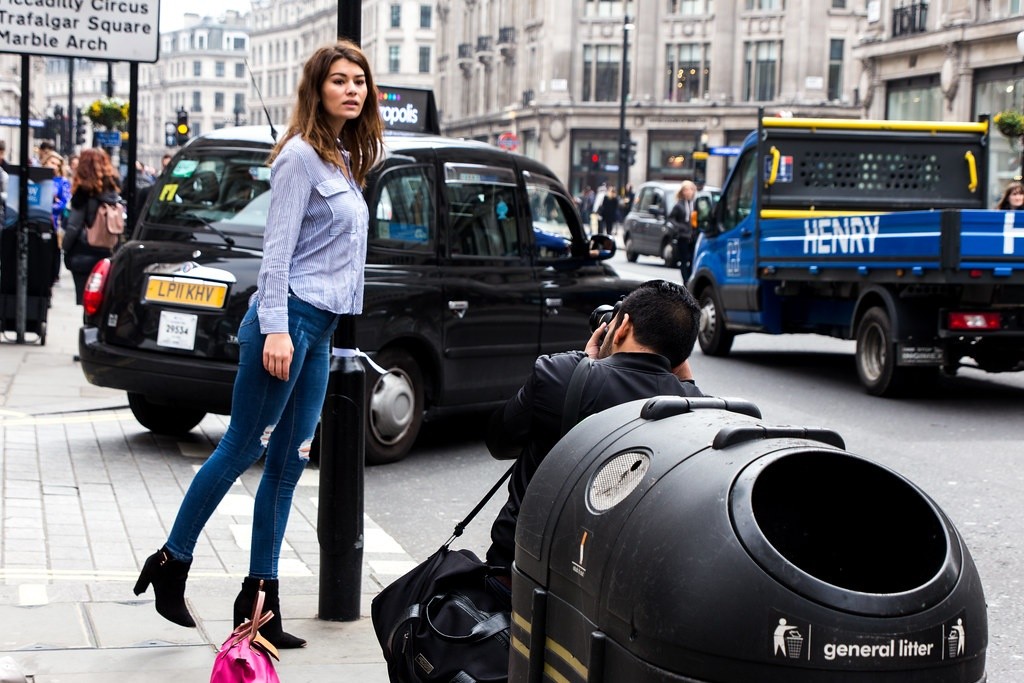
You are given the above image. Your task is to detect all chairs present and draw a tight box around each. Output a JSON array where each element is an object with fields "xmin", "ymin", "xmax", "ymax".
[{"xmin": 449, "ymin": 198, "xmax": 495, "ymax": 255}]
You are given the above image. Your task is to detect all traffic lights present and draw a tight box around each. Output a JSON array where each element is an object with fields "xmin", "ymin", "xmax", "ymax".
[
  {"xmin": 628, "ymin": 140, "xmax": 638, "ymax": 166},
  {"xmin": 175, "ymin": 111, "xmax": 191, "ymax": 146},
  {"xmin": 591, "ymin": 153, "xmax": 600, "ymax": 170},
  {"xmin": 619, "ymin": 141, "xmax": 629, "ymax": 163}
]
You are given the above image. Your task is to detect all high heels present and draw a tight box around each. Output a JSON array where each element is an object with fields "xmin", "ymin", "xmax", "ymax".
[
  {"xmin": 233, "ymin": 577, "xmax": 307, "ymax": 649},
  {"xmin": 133, "ymin": 546, "xmax": 196, "ymax": 628}
]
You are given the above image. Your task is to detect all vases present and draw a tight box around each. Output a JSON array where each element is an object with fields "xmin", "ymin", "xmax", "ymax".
[{"xmin": 1000, "ymin": 125, "xmax": 1023, "ymax": 137}]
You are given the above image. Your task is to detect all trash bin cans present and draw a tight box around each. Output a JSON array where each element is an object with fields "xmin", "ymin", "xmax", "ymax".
[{"xmin": 508, "ymin": 395, "xmax": 989, "ymax": 683}]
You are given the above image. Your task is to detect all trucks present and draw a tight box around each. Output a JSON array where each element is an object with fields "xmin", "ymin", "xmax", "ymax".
[{"xmin": 686, "ymin": 106, "xmax": 1024, "ymax": 397}]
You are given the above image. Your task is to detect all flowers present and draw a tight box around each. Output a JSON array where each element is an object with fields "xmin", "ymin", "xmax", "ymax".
[
  {"xmin": 85, "ymin": 97, "xmax": 129, "ymax": 129},
  {"xmin": 993, "ymin": 109, "xmax": 1024, "ymax": 150}
]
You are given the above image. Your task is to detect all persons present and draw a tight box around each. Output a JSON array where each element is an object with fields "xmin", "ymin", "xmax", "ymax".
[
  {"xmin": 670, "ymin": 180, "xmax": 700, "ymax": 285},
  {"xmin": 134, "ymin": 39, "xmax": 386, "ymax": 649},
  {"xmin": 484, "ymin": 278, "xmax": 703, "ymax": 587},
  {"xmin": 527, "ymin": 182, "xmax": 634, "ymax": 236},
  {"xmin": 998, "ymin": 182, "xmax": 1024, "ymax": 210},
  {"xmin": 1, "ymin": 139, "xmax": 174, "ymax": 360}
]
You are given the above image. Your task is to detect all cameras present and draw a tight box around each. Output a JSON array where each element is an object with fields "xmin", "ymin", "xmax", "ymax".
[{"xmin": 588, "ymin": 299, "xmax": 624, "ymax": 341}]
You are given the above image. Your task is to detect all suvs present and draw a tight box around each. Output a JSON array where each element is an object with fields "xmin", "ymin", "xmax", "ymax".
[
  {"xmin": 621, "ymin": 180, "xmax": 721, "ymax": 267},
  {"xmin": 75, "ymin": 124, "xmax": 647, "ymax": 467}
]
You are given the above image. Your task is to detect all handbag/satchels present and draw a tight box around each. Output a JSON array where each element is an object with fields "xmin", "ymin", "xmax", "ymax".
[
  {"xmin": 210, "ymin": 590, "xmax": 280, "ymax": 683},
  {"xmin": 371, "ymin": 545, "xmax": 512, "ymax": 683}
]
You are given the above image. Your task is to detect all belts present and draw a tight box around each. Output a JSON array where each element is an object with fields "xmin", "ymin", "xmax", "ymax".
[{"xmin": 493, "ymin": 573, "xmax": 512, "ymax": 592}]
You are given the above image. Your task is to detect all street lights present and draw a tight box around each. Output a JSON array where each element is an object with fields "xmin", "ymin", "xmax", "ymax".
[{"xmin": 614, "ymin": 14, "xmax": 635, "ymax": 224}]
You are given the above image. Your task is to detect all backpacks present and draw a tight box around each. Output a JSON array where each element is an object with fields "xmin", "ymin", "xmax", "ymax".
[{"xmin": 84, "ymin": 184, "xmax": 127, "ymax": 249}]
